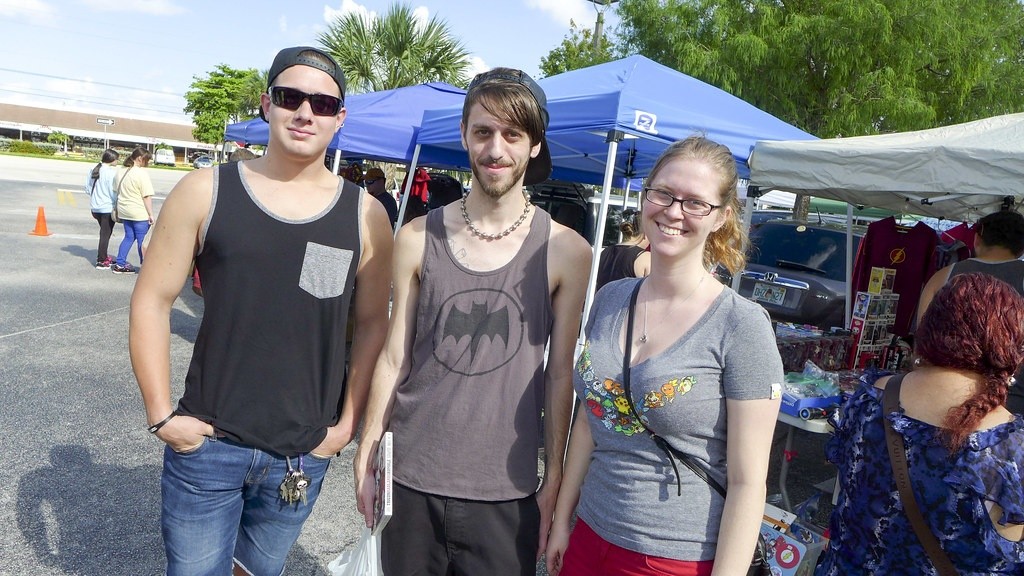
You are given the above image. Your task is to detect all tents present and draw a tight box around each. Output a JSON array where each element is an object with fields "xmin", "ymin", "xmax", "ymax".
[
  {"xmin": 220, "ymin": 82, "xmax": 643, "ymax": 245},
  {"xmin": 394, "ymin": 55, "xmax": 854, "ymax": 455},
  {"xmin": 729, "ymin": 112, "xmax": 1024, "ymax": 293}
]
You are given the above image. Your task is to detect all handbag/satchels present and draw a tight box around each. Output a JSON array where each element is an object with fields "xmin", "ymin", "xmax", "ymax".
[
  {"xmin": 747, "ymin": 533, "xmax": 773, "ymax": 576},
  {"xmin": 141, "ymin": 224, "xmax": 156, "ymax": 260},
  {"xmin": 111, "ymin": 204, "xmax": 124, "ymax": 223}
]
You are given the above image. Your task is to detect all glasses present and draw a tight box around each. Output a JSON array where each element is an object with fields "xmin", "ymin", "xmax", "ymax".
[
  {"xmin": 644, "ymin": 186, "xmax": 722, "ymax": 216},
  {"xmin": 365, "ymin": 178, "xmax": 379, "ymax": 185},
  {"xmin": 267, "ymin": 85, "xmax": 344, "ymax": 118}
]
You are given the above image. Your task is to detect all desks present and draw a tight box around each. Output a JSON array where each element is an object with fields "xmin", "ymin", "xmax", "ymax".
[{"xmin": 777, "ymin": 409, "xmax": 841, "ymax": 514}]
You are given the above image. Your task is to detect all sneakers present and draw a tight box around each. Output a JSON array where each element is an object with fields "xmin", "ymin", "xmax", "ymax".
[
  {"xmin": 96, "ymin": 259, "xmax": 111, "ymax": 270},
  {"xmin": 106, "ymin": 255, "xmax": 117, "ymax": 265},
  {"xmin": 112, "ymin": 265, "xmax": 136, "ymax": 274}
]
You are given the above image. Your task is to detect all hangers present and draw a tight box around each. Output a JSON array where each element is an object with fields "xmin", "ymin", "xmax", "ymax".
[{"xmin": 863, "ymin": 200, "xmax": 985, "ymax": 254}]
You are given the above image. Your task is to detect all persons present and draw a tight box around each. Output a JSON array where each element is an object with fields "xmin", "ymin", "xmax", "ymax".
[
  {"xmin": 229, "ymin": 148, "xmax": 256, "ymax": 161},
  {"xmin": 343, "ymin": 164, "xmax": 426, "ymax": 227},
  {"xmin": 113, "ymin": 148, "xmax": 154, "ymax": 274},
  {"xmin": 129, "ymin": 47, "xmax": 395, "ymax": 576},
  {"xmin": 545, "ymin": 136, "xmax": 784, "ymax": 576},
  {"xmin": 352, "ymin": 67, "xmax": 593, "ymax": 576},
  {"xmin": 84, "ymin": 149, "xmax": 121, "ymax": 270},
  {"xmin": 813, "ymin": 273, "xmax": 1024, "ymax": 576},
  {"xmin": 596, "ymin": 209, "xmax": 651, "ymax": 293},
  {"xmin": 917, "ymin": 211, "xmax": 1024, "ymax": 333}
]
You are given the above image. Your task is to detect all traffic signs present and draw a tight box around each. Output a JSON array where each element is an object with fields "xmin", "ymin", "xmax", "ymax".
[{"xmin": 97, "ymin": 118, "xmax": 115, "ymax": 125}]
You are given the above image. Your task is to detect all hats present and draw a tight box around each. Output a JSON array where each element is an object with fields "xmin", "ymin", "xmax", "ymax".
[
  {"xmin": 469, "ymin": 69, "xmax": 552, "ymax": 187},
  {"xmin": 361, "ymin": 168, "xmax": 384, "ymax": 179},
  {"xmin": 259, "ymin": 46, "xmax": 346, "ymax": 124}
]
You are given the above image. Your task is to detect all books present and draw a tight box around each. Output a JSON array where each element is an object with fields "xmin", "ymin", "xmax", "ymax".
[{"xmin": 370, "ymin": 432, "xmax": 393, "ymax": 537}]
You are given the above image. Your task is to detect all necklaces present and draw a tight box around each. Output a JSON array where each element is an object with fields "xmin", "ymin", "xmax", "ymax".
[
  {"xmin": 461, "ymin": 189, "xmax": 530, "ymax": 239},
  {"xmin": 640, "ymin": 272, "xmax": 707, "ymax": 343}
]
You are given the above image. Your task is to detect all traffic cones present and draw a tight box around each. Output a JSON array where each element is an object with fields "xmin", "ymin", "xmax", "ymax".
[{"xmin": 28, "ymin": 206, "xmax": 52, "ymax": 236}]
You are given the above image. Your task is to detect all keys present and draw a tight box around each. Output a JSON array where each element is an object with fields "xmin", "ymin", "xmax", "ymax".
[{"xmin": 281, "ymin": 469, "xmax": 311, "ymax": 507}]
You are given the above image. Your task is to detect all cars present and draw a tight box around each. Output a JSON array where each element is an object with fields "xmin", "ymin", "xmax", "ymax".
[
  {"xmin": 155, "ymin": 149, "xmax": 175, "ymax": 167},
  {"xmin": 715, "ymin": 219, "xmax": 907, "ymax": 332},
  {"xmin": 193, "ymin": 157, "xmax": 214, "ymax": 169},
  {"xmin": 363, "ymin": 171, "xmax": 399, "ymax": 211},
  {"xmin": 750, "ymin": 210, "xmax": 848, "ymax": 231}
]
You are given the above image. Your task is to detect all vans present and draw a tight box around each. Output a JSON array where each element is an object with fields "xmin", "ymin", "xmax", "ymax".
[{"xmin": 526, "ymin": 180, "xmax": 638, "ymax": 253}]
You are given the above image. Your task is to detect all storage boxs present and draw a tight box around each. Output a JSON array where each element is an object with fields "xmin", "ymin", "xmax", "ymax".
[
  {"xmin": 759, "ymin": 501, "xmax": 830, "ymax": 576},
  {"xmin": 779, "ymin": 266, "xmax": 902, "ymax": 417}
]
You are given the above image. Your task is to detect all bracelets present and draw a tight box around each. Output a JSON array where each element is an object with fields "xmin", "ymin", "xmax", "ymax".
[{"xmin": 147, "ymin": 411, "xmax": 176, "ymax": 434}]
